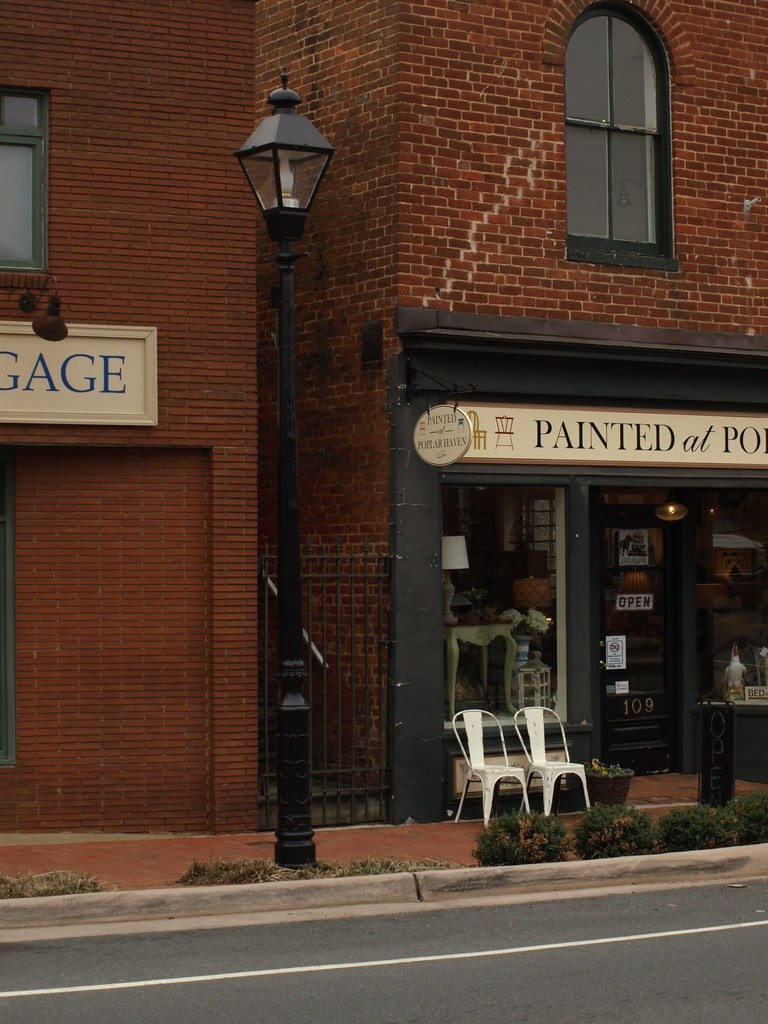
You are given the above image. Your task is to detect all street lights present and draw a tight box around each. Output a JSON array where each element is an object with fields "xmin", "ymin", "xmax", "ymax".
[{"xmin": 235, "ymin": 71, "xmax": 339, "ymax": 867}]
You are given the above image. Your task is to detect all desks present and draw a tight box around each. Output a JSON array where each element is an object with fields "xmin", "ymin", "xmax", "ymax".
[{"xmin": 442, "ymin": 624, "xmax": 518, "ymax": 721}]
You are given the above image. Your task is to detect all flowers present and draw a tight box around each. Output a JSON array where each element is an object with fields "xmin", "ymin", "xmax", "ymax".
[
  {"xmin": 495, "ymin": 607, "xmax": 555, "ymax": 637},
  {"xmin": 583, "ymin": 752, "xmax": 628, "ymax": 777}
]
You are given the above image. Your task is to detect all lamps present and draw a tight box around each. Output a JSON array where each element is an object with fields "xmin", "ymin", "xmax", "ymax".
[
  {"xmin": 511, "ymin": 577, "xmax": 552, "ymax": 611},
  {"xmin": 441, "ymin": 536, "xmax": 470, "ymax": 626},
  {"xmin": 654, "ymin": 487, "xmax": 688, "ymax": 522},
  {"xmin": 19, "ymin": 276, "xmax": 69, "ymax": 341},
  {"xmin": 617, "ymin": 570, "xmax": 655, "ymax": 630}
]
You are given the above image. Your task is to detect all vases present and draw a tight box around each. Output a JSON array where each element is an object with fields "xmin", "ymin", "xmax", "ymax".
[
  {"xmin": 513, "ymin": 635, "xmax": 534, "ymax": 671},
  {"xmin": 576, "ymin": 769, "xmax": 636, "ymax": 807}
]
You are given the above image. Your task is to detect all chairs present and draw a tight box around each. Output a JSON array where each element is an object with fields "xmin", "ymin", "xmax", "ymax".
[
  {"xmin": 513, "ymin": 706, "xmax": 591, "ymax": 818},
  {"xmin": 452, "ymin": 709, "xmax": 532, "ymax": 830}
]
